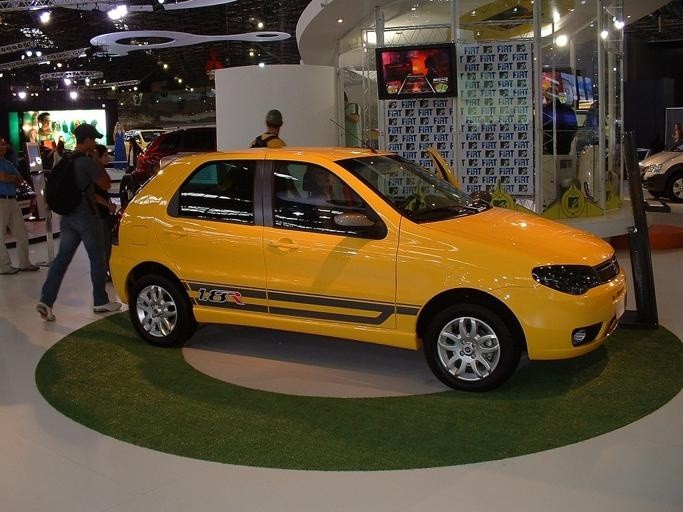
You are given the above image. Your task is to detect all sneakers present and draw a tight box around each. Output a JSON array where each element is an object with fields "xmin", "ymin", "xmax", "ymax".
[
  {"xmin": 0, "ymin": 268, "xmax": 21, "ymax": 274},
  {"xmin": 36, "ymin": 301, "xmax": 56, "ymax": 321},
  {"xmin": 94, "ymin": 302, "xmax": 122, "ymax": 313},
  {"xmin": 19, "ymin": 264, "xmax": 40, "ymax": 271}
]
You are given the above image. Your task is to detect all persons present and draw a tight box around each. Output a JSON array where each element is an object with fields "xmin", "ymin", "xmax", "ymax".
[
  {"xmin": 0, "ymin": 121, "xmax": 144, "ymax": 320},
  {"xmin": 543, "ymin": 87, "xmax": 577, "ymax": 155},
  {"xmin": 672, "ymin": 123, "xmax": 683, "ymax": 142},
  {"xmin": 24, "ymin": 111, "xmax": 96, "ymax": 150},
  {"xmin": 247, "ymin": 109, "xmax": 303, "ymax": 199},
  {"xmin": 303, "ymin": 167, "xmax": 343, "ymax": 231}
]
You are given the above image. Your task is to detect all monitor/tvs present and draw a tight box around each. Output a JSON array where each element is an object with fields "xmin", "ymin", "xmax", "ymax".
[{"xmin": 376, "ymin": 43, "xmax": 458, "ymax": 100}]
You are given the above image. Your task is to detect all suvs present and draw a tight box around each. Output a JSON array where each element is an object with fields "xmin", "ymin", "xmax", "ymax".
[{"xmin": 136, "ymin": 125, "xmax": 217, "ymax": 179}]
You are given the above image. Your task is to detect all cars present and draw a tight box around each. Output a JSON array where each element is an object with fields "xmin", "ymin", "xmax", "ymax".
[
  {"xmin": 105, "ymin": 146, "xmax": 625, "ymax": 393},
  {"xmin": 124, "ymin": 129, "xmax": 168, "ymax": 158},
  {"xmin": 638, "ymin": 137, "xmax": 683, "ymax": 202},
  {"xmin": 14, "ymin": 179, "xmax": 37, "ymax": 217}
]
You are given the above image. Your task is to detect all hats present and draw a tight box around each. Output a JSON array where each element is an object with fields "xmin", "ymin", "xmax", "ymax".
[
  {"xmin": 266, "ymin": 110, "xmax": 283, "ymax": 126},
  {"xmin": 74, "ymin": 123, "xmax": 103, "ymax": 139}
]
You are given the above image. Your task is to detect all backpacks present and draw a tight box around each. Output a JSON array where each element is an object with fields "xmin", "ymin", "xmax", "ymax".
[
  {"xmin": 45, "ymin": 151, "xmax": 94, "ymax": 215},
  {"xmin": 251, "ymin": 135, "xmax": 279, "ymax": 147}
]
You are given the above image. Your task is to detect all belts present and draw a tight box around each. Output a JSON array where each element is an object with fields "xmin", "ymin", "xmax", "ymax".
[{"xmin": 0, "ymin": 194, "xmax": 16, "ymax": 200}]
